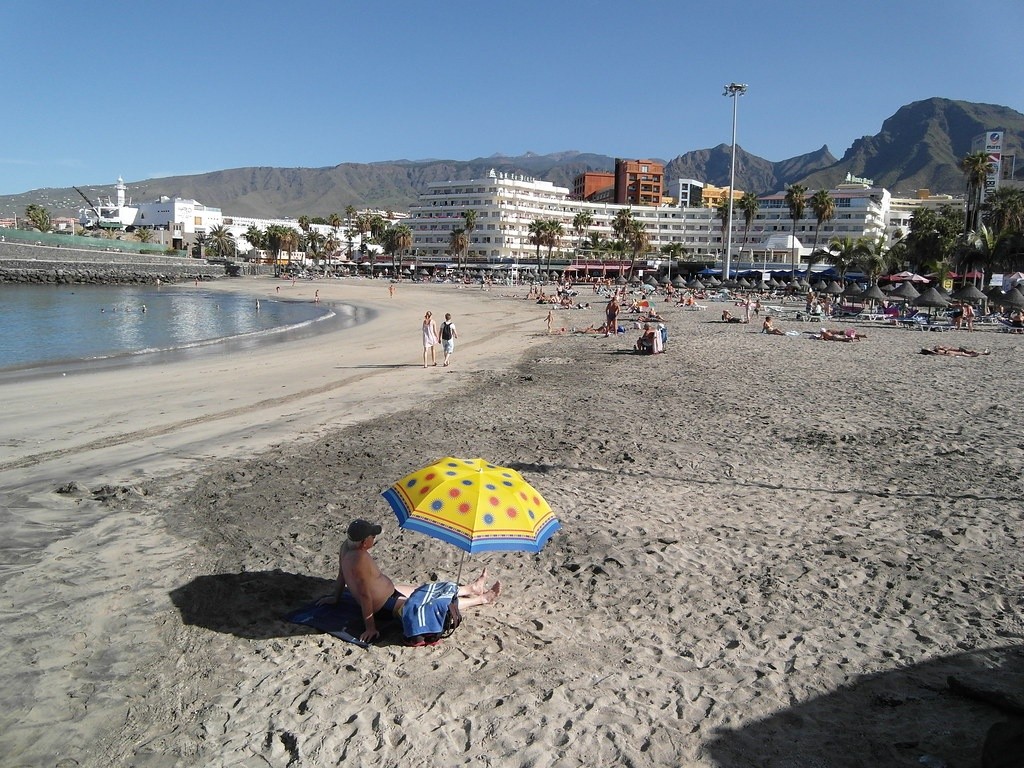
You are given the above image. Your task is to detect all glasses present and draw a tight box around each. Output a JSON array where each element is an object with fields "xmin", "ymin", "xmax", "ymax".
[{"xmin": 368, "ymin": 535, "xmax": 376, "ymax": 539}]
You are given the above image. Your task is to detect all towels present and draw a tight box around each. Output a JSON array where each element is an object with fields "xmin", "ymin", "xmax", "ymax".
[
  {"xmin": 784, "ymin": 329, "xmax": 800, "ymax": 337},
  {"xmin": 402, "ymin": 582, "xmax": 458, "ymax": 637},
  {"xmin": 279, "ymin": 596, "xmax": 399, "ymax": 647},
  {"xmin": 652, "ymin": 330, "xmax": 663, "ymax": 353}
]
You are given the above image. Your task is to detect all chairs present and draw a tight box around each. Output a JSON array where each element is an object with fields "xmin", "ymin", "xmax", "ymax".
[
  {"xmin": 798, "ymin": 305, "xmax": 1024, "ymax": 334},
  {"xmin": 639, "ymin": 330, "xmax": 668, "ymax": 353},
  {"xmin": 722, "ymin": 315, "xmax": 739, "ymax": 323}
]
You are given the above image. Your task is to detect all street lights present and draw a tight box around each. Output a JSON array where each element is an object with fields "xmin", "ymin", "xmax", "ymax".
[
  {"xmin": 231, "ymin": 237, "xmax": 237, "ymax": 262},
  {"xmin": 72, "ymin": 218, "xmax": 75, "ymax": 235},
  {"xmin": 416, "ymin": 248, "xmax": 419, "ymax": 272},
  {"xmin": 723, "ymin": 82, "xmax": 749, "ymax": 281},
  {"xmin": 13, "ymin": 212, "xmax": 17, "ymax": 230},
  {"xmin": 669, "ymin": 250, "xmax": 674, "ymax": 279},
  {"xmin": 160, "ymin": 228, "xmax": 164, "ymax": 245}
]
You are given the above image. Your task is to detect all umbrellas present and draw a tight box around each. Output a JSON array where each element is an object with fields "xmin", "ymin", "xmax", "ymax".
[
  {"xmin": 881, "ymin": 270, "xmax": 931, "ymax": 306},
  {"xmin": 767, "ymin": 269, "xmax": 809, "ymax": 295},
  {"xmin": 879, "ymin": 284, "xmax": 895, "ymax": 292},
  {"xmin": 661, "ymin": 269, "xmax": 722, "ymax": 292},
  {"xmin": 403, "ymin": 269, "xmax": 559, "ymax": 281},
  {"xmin": 986, "ymin": 283, "xmax": 1024, "ymax": 312},
  {"xmin": 813, "ymin": 269, "xmax": 843, "ymax": 301},
  {"xmin": 616, "ymin": 275, "xmax": 658, "ymax": 287},
  {"xmin": 926, "ymin": 271, "xmax": 988, "ymax": 304},
  {"xmin": 859, "ymin": 283, "xmax": 887, "ymax": 313},
  {"xmin": 381, "ymin": 455, "xmax": 560, "ymax": 598},
  {"xmin": 912, "ymin": 287, "xmax": 952, "ymax": 314},
  {"xmin": 723, "ymin": 269, "xmax": 770, "ymax": 293},
  {"xmin": 842, "ymin": 282, "xmax": 862, "ymax": 307},
  {"xmin": 284, "ymin": 260, "xmax": 399, "ymax": 276}
]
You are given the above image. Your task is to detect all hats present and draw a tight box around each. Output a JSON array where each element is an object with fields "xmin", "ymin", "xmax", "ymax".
[{"xmin": 346, "ymin": 519, "xmax": 382, "ymax": 541}]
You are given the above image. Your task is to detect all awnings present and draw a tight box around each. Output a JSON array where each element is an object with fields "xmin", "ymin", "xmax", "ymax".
[{"xmin": 572, "ymin": 265, "xmax": 631, "ymax": 270}]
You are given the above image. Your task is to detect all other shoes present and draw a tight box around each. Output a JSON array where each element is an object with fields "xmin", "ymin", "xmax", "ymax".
[
  {"xmin": 399, "ymin": 631, "xmax": 425, "ymax": 647},
  {"xmin": 422, "ymin": 633, "xmax": 439, "ymax": 643},
  {"xmin": 443, "ymin": 361, "xmax": 448, "ymax": 367}
]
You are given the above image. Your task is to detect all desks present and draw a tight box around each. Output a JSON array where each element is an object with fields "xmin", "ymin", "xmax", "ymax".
[{"xmin": 691, "ymin": 302, "xmax": 708, "ymax": 311}]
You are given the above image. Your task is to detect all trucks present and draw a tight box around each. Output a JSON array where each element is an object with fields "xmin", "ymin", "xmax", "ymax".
[{"xmin": 72, "ymin": 186, "xmax": 123, "ymax": 231}]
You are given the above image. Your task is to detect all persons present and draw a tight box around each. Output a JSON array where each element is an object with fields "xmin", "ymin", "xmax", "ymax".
[
  {"xmin": 761, "ymin": 293, "xmax": 1024, "ymax": 358},
  {"xmin": 480, "ymin": 274, "xmax": 613, "ymax": 334},
  {"xmin": 604, "ymin": 283, "xmax": 761, "ymax": 354},
  {"xmin": 315, "ymin": 519, "xmax": 501, "ymax": 642},
  {"xmin": 422, "ymin": 311, "xmax": 438, "ymax": 368},
  {"xmin": 438, "ymin": 313, "xmax": 458, "ymax": 366},
  {"xmin": 104, "ymin": 272, "xmax": 395, "ymax": 314}
]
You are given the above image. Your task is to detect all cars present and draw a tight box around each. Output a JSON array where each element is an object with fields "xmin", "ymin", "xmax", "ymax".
[
  {"xmin": 492, "ymin": 279, "xmax": 504, "ymax": 283},
  {"xmin": 436, "ymin": 277, "xmax": 445, "ymax": 282}
]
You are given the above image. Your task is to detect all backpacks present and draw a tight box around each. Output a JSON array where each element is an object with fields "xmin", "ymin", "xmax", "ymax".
[{"xmin": 442, "ymin": 322, "xmax": 452, "ymax": 340}]
